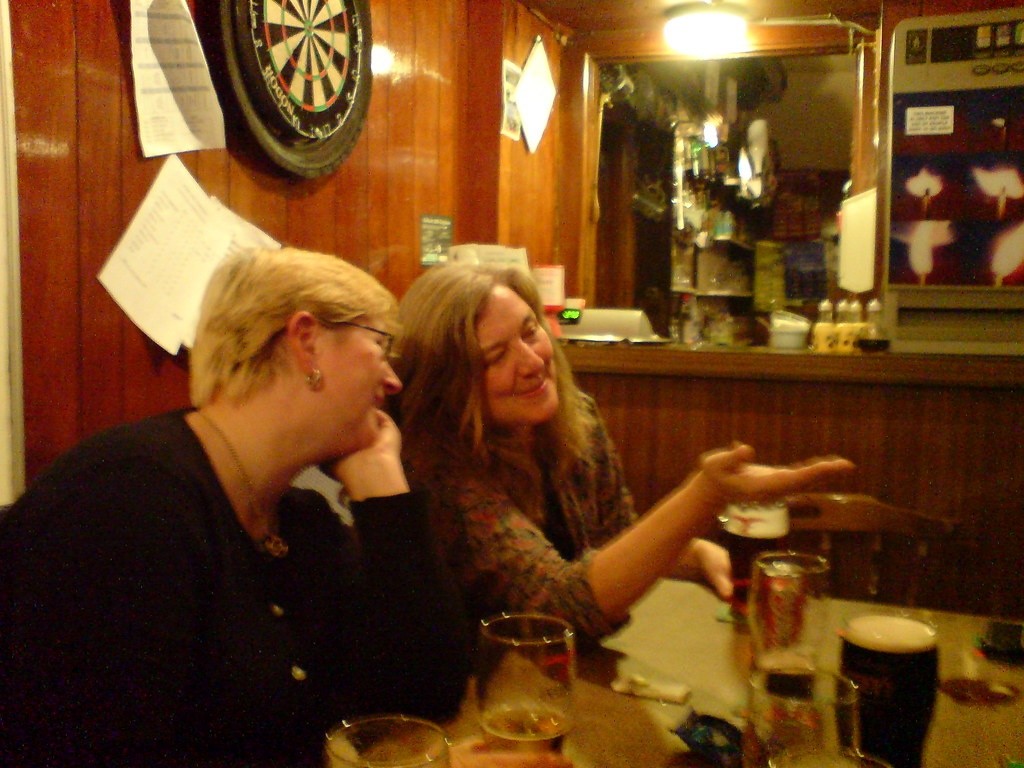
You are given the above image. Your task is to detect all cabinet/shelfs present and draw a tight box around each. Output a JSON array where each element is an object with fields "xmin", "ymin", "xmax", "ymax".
[{"xmin": 635, "ymin": 133, "xmax": 754, "ymax": 335}]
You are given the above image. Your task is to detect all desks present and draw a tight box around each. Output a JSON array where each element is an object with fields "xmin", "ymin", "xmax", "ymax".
[{"xmin": 341, "ymin": 581, "xmax": 1024, "ymax": 768}]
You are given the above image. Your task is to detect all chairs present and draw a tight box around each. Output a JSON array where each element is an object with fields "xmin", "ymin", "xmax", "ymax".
[{"xmin": 729, "ymin": 494, "xmax": 962, "ymax": 609}]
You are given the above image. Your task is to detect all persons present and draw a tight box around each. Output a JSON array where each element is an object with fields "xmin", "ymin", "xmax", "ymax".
[
  {"xmin": 1, "ymin": 247, "xmax": 468, "ymax": 768},
  {"xmin": 390, "ymin": 262, "xmax": 855, "ymax": 642}
]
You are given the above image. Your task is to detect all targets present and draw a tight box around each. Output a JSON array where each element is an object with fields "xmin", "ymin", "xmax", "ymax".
[{"xmin": 210, "ymin": 0, "xmax": 374, "ymax": 182}]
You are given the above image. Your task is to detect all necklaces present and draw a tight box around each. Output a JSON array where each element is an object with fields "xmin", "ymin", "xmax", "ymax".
[{"xmin": 199, "ymin": 408, "xmax": 289, "ymax": 557}]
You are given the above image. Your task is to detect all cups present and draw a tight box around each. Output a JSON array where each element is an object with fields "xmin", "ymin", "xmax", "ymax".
[
  {"xmin": 748, "ymin": 668, "xmax": 860, "ymax": 767},
  {"xmin": 752, "ymin": 551, "xmax": 830, "ymax": 669},
  {"xmin": 724, "ymin": 500, "xmax": 790, "ymax": 623},
  {"xmin": 835, "ymin": 606, "xmax": 941, "ymax": 768},
  {"xmin": 474, "ymin": 612, "xmax": 576, "ymax": 755},
  {"xmin": 323, "ymin": 714, "xmax": 460, "ymax": 768}
]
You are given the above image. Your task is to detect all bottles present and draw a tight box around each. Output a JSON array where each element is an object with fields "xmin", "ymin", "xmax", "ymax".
[{"xmin": 809, "ymin": 297, "xmax": 893, "ymax": 354}]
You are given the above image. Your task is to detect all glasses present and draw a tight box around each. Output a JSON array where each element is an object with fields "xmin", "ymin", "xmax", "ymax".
[{"xmin": 272, "ymin": 320, "xmax": 394, "ymax": 357}]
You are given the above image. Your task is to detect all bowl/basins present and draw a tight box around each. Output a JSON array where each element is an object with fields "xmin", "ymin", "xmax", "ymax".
[{"xmin": 768, "ymin": 309, "xmax": 811, "ymax": 349}]
